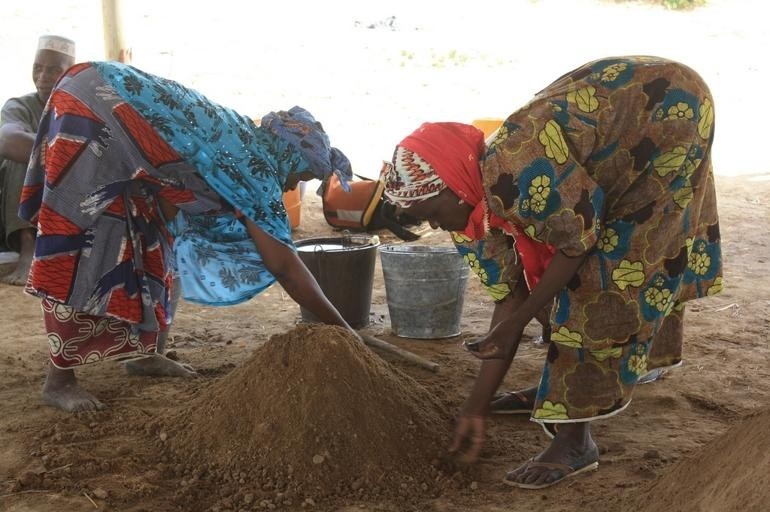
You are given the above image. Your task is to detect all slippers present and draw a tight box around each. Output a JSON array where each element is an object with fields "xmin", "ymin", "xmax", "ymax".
[
  {"xmin": 504, "ymin": 455, "xmax": 598, "ymax": 490},
  {"xmin": 489, "ymin": 390, "xmax": 534, "ymax": 415}
]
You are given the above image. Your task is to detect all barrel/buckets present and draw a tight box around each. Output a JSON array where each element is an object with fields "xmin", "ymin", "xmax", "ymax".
[
  {"xmin": 291, "ymin": 231, "xmax": 380, "ymax": 323},
  {"xmin": 379, "ymin": 245, "xmax": 470, "ymax": 339}
]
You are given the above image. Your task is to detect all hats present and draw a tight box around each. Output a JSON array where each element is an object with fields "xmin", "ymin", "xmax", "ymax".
[
  {"xmin": 37, "ymin": 36, "xmax": 76, "ymax": 58},
  {"xmin": 379, "ymin": 145, "xmax": 447, "ymax": 217}
]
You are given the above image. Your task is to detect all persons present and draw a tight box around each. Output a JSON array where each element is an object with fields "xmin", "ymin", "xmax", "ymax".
[
  {"xmin": 379, "ymin": 56, "xmax": 724, "ymax": 489},
  {"xmin": 0, "ymin": 35, "xmax": 75, "ymax": 285},
  {"xmin": 17, "ymin": 61, "xmax": 363, "ymax": 413}
]
